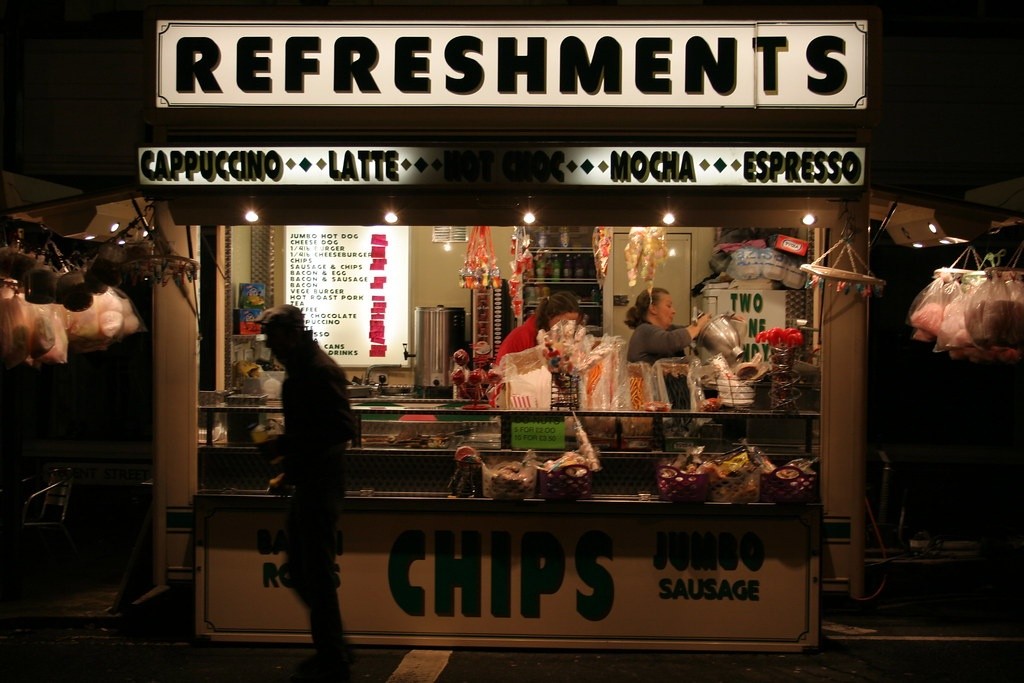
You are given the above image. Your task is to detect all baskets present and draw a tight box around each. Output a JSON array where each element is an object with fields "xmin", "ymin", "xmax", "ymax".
[
  {"xmin": 655, "ymin": 463, "xmax": 817, "ymax": 503},
  {"xmin": 447, "ymin": 451, "xmax": 592, "ymax": 499},
  {"xmin": 551, "ymin": 372, "xmax": 580, "ymax": 410}
]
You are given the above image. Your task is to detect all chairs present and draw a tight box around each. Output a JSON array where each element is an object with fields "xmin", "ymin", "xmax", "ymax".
[{"xmin": 16, "ymin": 468, "xmax": 80, "ymax": 561}]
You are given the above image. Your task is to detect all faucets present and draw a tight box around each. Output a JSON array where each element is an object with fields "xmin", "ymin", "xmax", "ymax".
[{"xmin": 403, "ymin": 343, "xmax": 416, "ymax": 360}]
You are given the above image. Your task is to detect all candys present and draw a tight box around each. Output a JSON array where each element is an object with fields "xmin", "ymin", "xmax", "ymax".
[
  {"xmin": 756, "ymin": 328, "xmax": 804, "ymax": 350},
  {"xmin": 541, "ymin": 336, "xmax": 573, "ymax": 378},
  {"xmin": 450, "ymin": 340, "xmax": 503, "ymax": 404}
]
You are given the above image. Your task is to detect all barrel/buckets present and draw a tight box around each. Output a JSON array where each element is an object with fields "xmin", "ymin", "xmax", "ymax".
[{"xmin": 411, "ymin": 305, "xmax": 465, "ymax": 400}]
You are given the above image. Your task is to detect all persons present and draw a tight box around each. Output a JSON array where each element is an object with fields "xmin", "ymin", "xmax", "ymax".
[
  {"xmin": 247, "ymin": 305, "xmax": 355, "ymax": 678},
  {"xmin": 624, "ymin": 287, "xmax": 713, "ymax": 410},
  {"xmin": 496, "ymin": 290, "xmax": 582, "ymax": 403}
]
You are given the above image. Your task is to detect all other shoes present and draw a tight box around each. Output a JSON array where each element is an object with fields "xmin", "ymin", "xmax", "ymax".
[{"xmin": 288, "ymin": 655, "xmax": 354, "ymax": 683}]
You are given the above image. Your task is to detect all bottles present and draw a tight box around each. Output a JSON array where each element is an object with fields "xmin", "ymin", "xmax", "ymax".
[
  {"xmin": 576, "ymin": 254, "xmax": 583, "ymax": 278},
  {"xmin": 552, "ymin": 254, "xmax": 560, "ymax": 278},
  {"xmin": 564, "ymin": 254, "xmax": 572, "ymax": 279},
  {"xmin": 537, "ymin": 254, "xmax": 545, "ymax": 278}
]
